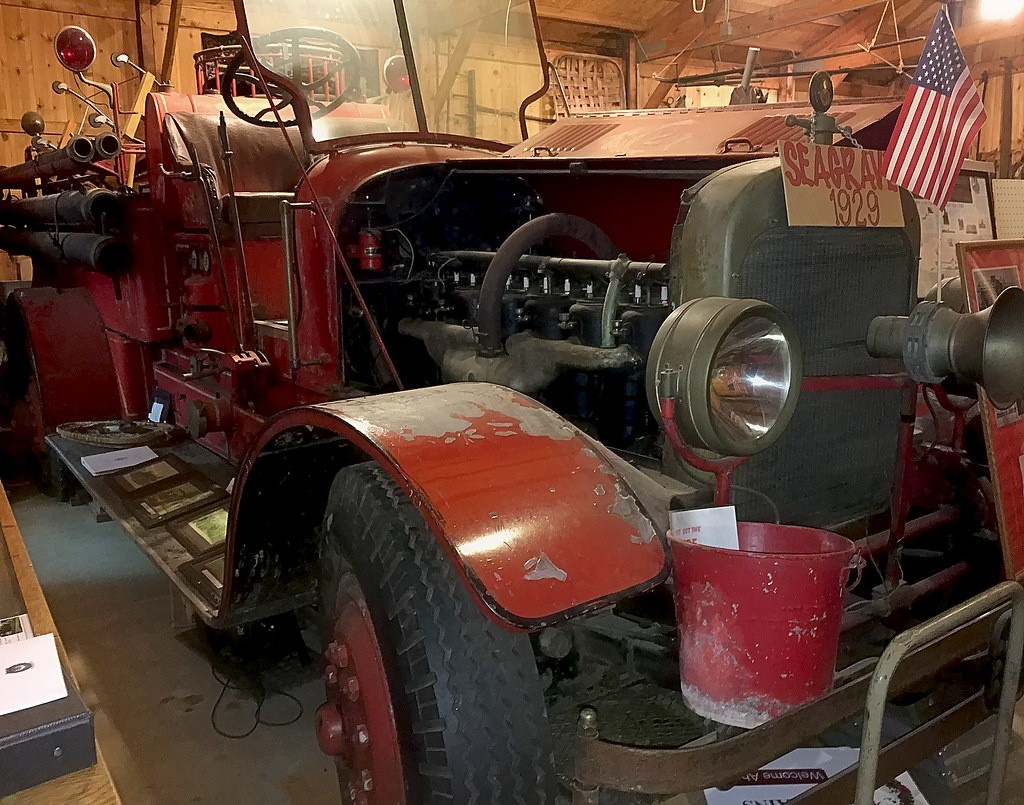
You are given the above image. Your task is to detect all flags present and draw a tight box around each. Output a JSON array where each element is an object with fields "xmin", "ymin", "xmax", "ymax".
[{"xmin": 882, "ymin": 8, "xmax": 988, "ymax": 212}]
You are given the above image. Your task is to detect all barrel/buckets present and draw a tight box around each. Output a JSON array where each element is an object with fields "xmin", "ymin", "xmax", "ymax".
[{"xmin": 665, "ymin": 522, "xmax": 867, "ymax": 730}]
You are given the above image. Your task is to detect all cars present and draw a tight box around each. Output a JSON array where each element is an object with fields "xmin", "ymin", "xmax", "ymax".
[{"xmin": 0, "ymin": 1, "xmax": 1024, "ymax": 805}]
